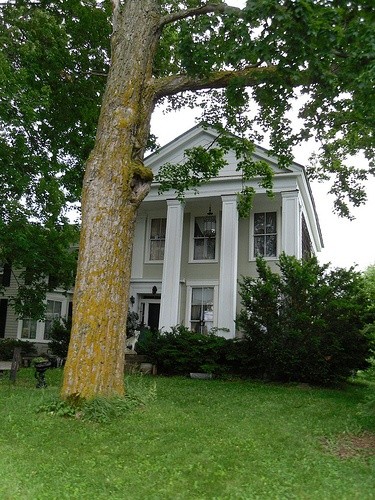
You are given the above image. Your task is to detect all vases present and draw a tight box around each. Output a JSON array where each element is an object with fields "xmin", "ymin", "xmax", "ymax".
[{"xmin": 189, "ymin": 372, "xmax": 212, "ymax": 379}]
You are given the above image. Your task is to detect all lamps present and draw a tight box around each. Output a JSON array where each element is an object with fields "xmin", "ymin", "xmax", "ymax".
[{"xmin": 130, "ymin": 295, "xmax": 135, "ymax": 307}]
